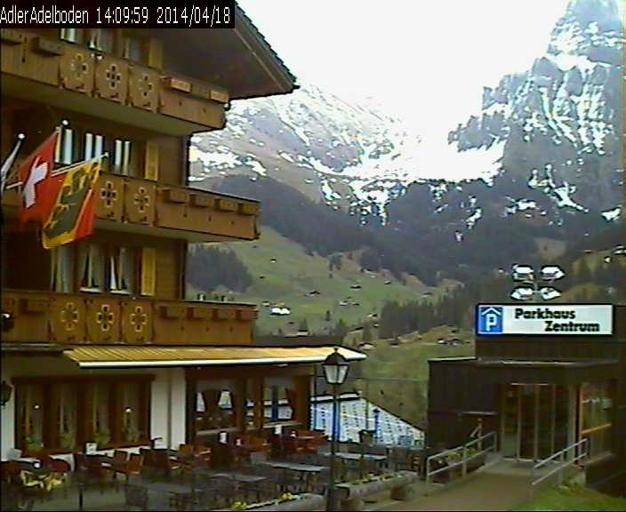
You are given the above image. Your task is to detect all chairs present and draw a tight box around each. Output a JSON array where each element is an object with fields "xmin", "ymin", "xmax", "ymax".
[{"xmin": 1, "ymin": 424, "xmax": 434, "ymax": 511}]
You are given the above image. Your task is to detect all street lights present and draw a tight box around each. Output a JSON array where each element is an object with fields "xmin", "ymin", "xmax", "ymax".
[
  {"xmin": 321, "ymin": 348, "xmax": 349, "ymax": 512},
  {"xmin": 509, "ymin": 263, "xmax": 564, "ymax": 303}
]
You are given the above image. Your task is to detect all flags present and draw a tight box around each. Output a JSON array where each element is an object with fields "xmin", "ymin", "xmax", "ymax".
[{"xmin": 0, "ymin": 117, "xmax": 112, "ymax": 250}]
